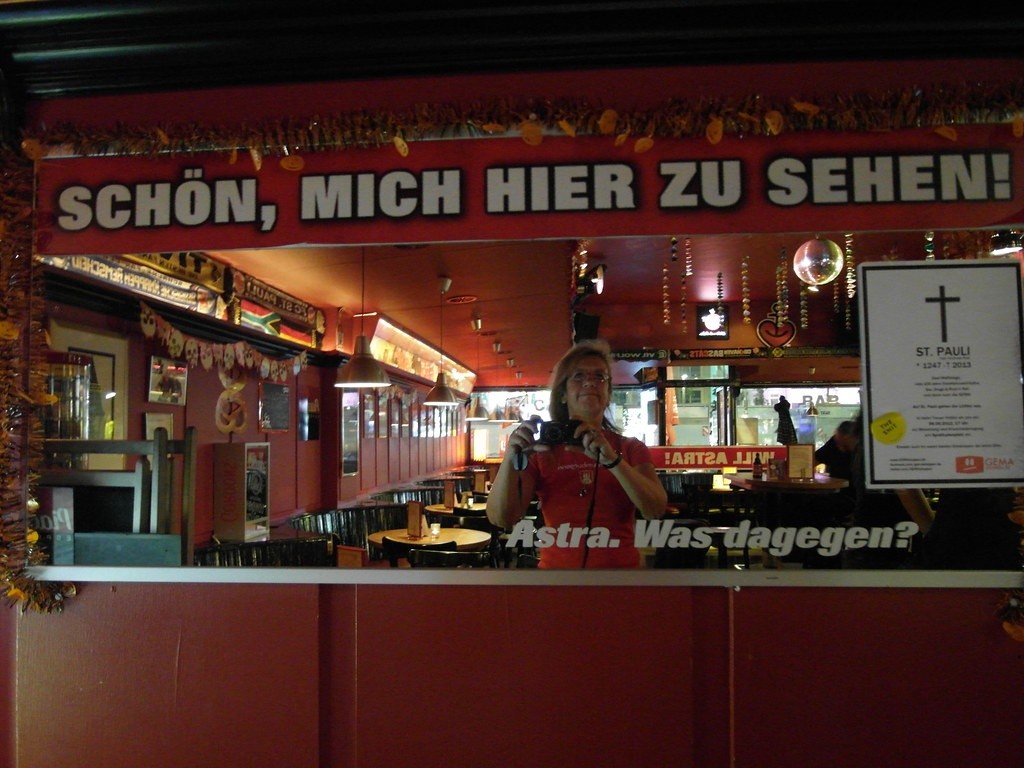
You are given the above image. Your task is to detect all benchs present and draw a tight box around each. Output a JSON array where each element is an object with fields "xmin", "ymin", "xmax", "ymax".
[
  {"xmin": 196, "ymin": 538, "xmax": 336, "ymax": 568},
  {"xmin": 414, "ymin": 478, "xmax": 472, "ymax": 492},
  {"xmin": 657, "ymin": 473, "xmax": 757, "ymax": 514},
  {"xmin": 442, "ymin": 466, "xmax": 488, "ymax": 476},
  {"xmin": 369, "ymin": 489, "xmax": 445, "ymax": 507},
  {"xmin": 287, "ymin": 503, "xmax": 429, "ymax": 560}
]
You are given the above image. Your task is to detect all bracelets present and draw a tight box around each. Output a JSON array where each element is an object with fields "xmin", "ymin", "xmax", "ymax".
[{"xmin": 603, "ymin": 453, "xmax": 622, "ymax": 469}]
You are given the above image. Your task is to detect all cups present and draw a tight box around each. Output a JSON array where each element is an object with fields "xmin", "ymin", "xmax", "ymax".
[
  {"xmin": 468, "ymin": 498, "xmax": 473, "ymax": 508},
  {"xmin": 431, "ymin": 523, "xmax": 440, "ymax": 538},
  {"xmin": 768, "ymin": 458, "xmax": 784, "ymax": 479}
]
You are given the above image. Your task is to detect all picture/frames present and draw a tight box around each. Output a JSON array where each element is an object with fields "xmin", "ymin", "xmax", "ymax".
[
  {"xmin": 342, "ymin": 388, "xmax": 359, "ymax": 478},
  {"xmin": 257, "ymin": 380, "xmax": 291, "ymax": 434},
  {"xmin": 49, "ymin": 319, "xmax": 130, "ymax": 471}
]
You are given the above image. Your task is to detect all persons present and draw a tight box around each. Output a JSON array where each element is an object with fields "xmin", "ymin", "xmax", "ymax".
[
  {"xmin": 814, "ymin": 420, "xmax": 865, "ymax": 498},
  {"xmin": 487, "ymin": 341, "xmax": 668, "ymax": 572},
  {"xmin": 854, "ymin": 410, "xmax": 936, "ymax": 570},
  {"xmin": 923, "ymin": 479, "xmax": 1021, "ymax": 569}
]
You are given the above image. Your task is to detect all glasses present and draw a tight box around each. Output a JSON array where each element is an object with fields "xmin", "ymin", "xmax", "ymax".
[{"xmin": 567, "ymin": 367, "xmax": 610, "ymax": 383}]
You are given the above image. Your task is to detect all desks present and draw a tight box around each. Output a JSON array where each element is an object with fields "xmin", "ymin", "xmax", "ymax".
[
  {"xmin": 425, "ymin": 503, "xmax": 488, "ymax": 516},
  {"xmin": 368, "ymin": 527, "xmax": 492, "ymax": 552},
  {"xmin": 725, "ymin": 473, "xmax": 849, "ymax": 568}
]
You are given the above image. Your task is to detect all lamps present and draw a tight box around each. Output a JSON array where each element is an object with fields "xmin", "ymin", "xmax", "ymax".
[
  {"xmin": 423, "ymin": 277, "xmax": 459, "ymax": 407},
  {"xmin": 487, "ymin": 342, "xmax": 522, "ymax": 424},
  {"xmin": 470, "ymin": 319, "xmax": 490, "ymax": 422},
  {"xmin": 334, "ymin": 246, "xmax": 392, "ymax": 389},
  {"xmin": 806, "ymin": 403, "xmax": 819, "ymax": 417}
]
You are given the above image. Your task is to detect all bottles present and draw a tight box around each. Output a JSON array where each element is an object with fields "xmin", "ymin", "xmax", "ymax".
[
  {"xmin": 753, "ymin": 453, "xmax": 762, "ymax": 478},
  {"xmin": 513, "ymin": 400, "xmax": 519, "ymax": 417}
]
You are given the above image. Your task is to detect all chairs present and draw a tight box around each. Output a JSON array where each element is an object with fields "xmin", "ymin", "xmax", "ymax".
[{"xmin": 383, "ymin": 491, "xmax": 543, "ymax": 569}]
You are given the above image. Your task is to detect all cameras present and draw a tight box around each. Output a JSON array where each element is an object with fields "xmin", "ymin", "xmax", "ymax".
[{"xmin": 542, "ymin": 420, "xmax": 584, "ymax": 445}]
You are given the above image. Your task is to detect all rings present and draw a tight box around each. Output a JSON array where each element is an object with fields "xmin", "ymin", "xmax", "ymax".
[{"xmin": 515, "ymin": 427, "xmax": 521, "ymax": 435}]
